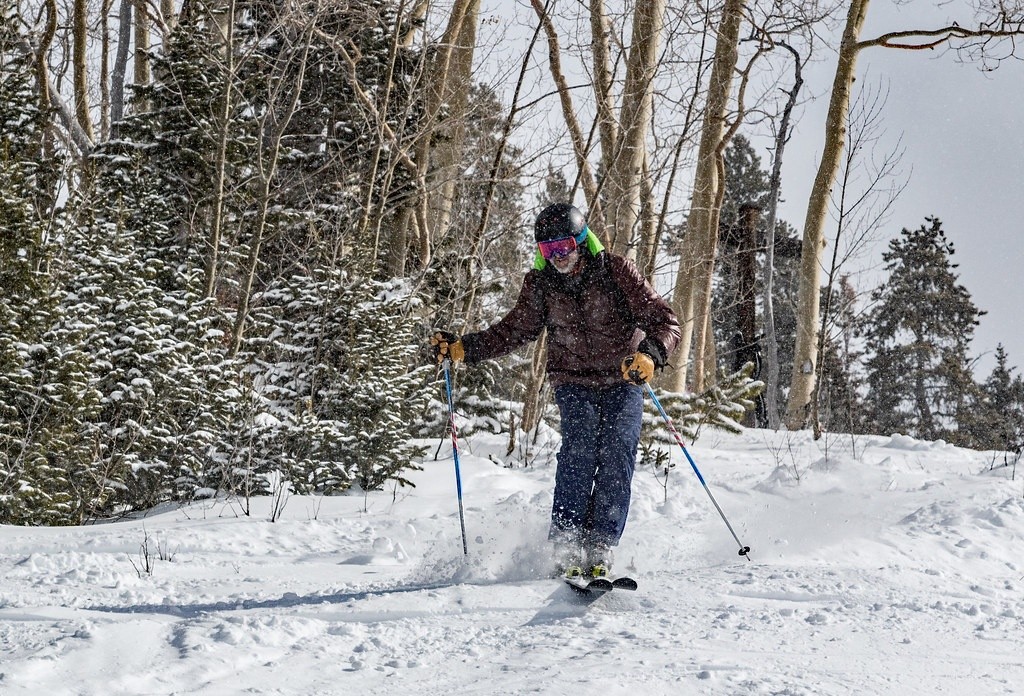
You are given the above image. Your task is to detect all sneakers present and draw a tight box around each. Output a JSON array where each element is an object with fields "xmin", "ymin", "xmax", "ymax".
[
  {"xmin": 567, "ymin": 566, "xmax": 581, "ymax": 577},
  {"xmin": 591, "ymin": 564, "xmax": 607, "ymax": 577}
]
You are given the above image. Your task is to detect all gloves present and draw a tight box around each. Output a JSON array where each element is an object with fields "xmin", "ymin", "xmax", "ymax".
[
  {"xmin": 431, "ymin": 331, "xmax": 464, "ymax": 362},
  {"xmin": 621, "ymin": 350, "xmax": 656, "ymax": 386}
]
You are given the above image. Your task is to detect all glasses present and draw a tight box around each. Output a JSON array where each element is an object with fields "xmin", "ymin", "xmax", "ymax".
[{"xmin": 537, "ymin": 237, "xmax": 576, "ymax": 259}]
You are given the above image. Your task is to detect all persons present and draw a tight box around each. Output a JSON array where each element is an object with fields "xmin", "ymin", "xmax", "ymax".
[{"xmin": 431, "ymin": 203, "xmax": 682, "ymax": 577}]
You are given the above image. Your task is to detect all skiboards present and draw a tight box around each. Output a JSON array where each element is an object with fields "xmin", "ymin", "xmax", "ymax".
[{"xmin": 551, "ymin": 566, "xmax": 638, "ymax": 593}]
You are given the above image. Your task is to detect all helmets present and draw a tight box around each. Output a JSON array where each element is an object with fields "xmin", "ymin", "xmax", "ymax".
[{"xmin": 535, "ymin": 203, "xmax": 588, "ymax": 247}]
